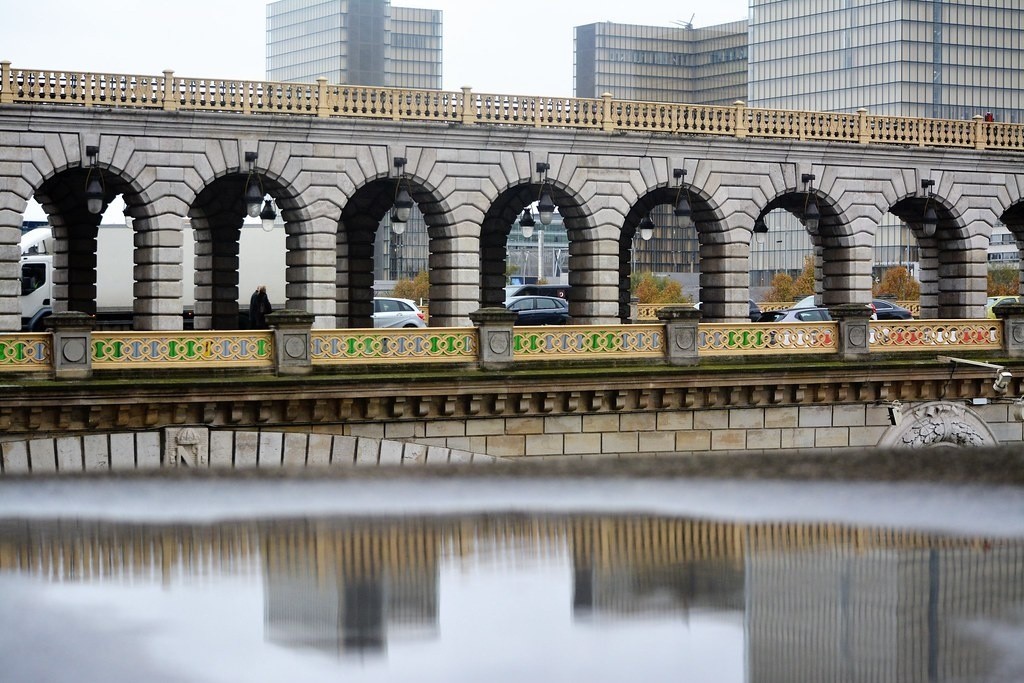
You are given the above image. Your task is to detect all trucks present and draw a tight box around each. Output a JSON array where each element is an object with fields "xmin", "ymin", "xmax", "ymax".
[
  {"xmin": 20, "ymin": 223, "xmax": 290, "ymax": 335},
  {"xmin": 505, "ymin": 285, "xmax": 571, "ymax": 303}
]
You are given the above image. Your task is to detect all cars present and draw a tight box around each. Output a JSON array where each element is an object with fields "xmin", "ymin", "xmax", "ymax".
[
  {"xmin": 692, "ymin": 299, "xmax": 761, "ymax": 323},
  {"xmin": 872, "ymin": 299, "xmax": 915, "ymax": 320},
  {"xmin": 987, "ymin": 296, "xmax": 1024, "ymax": 320},
  {"xmin": 506, "ymin": 295, "xmax": 569, "ymax": 326},
  {"xmin": 374, "ymin": 296, "xmax": 426, "ymax": 328},
  {"xmin": 755, "ymin": 308, "xmax": 835, "ymax": 323},
  {"xmin": 793, "ymin": 295, "xmax": 877, "ymax": 321}
]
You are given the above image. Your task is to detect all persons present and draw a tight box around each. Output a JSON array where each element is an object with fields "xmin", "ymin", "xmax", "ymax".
[{"xmin": 250, "ymin": 285, "xmax": 272, "ymax": 329}]
